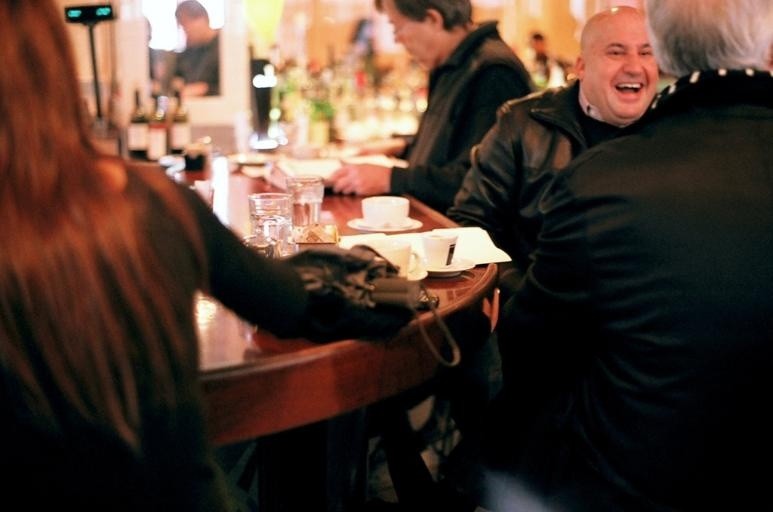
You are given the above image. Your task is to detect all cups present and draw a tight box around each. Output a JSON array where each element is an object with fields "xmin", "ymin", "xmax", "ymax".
[
  {"xmin": 368, "ymin": 242, "xmax": 422, "ymax": 276},
  {"xmin": 246, "ymin": 193, "xmax": 295, "ymax": 259},
  {"xmin": 360, "ymin": 194, "xmax": 411, "ymax": 230},
  {"xmin": 283, "ymin": 174, "xmax": 326, "ymax": 227},
  {"xmin": 417, "ymin": 229, "xmax": 462, "ymax": 269}
]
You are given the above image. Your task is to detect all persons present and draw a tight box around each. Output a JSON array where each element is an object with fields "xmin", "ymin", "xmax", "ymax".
[
  {"xmin": 0, "ymin": 0, "xmax": 308, "ymax": 512},
  {"xmin": 153, "ymin": 0, "xmax": 258, "ymax": 112},
  {"xmin": 315, "ymin": 0, "xmax": 537, "ymax": 218},
  {"xmin": 489, "ymin": 2, "xmax": 765, "ymax": 512},
  {"xmin": 441, "ymin": 6, "xmax": 658, "ymax": 278}
]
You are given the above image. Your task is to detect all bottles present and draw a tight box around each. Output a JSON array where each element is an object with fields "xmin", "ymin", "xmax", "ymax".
[
  {"xmin": 243, "ymin": 227, "xmax": 281, "ymax": 263},
  {"xmin": 128, "ymin": 83, "xmax": 191, "ymax": 160}
]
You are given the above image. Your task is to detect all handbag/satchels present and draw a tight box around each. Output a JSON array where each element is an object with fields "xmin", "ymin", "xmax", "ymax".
[{"xmin": 257, "ymin": 246, "xmax": 462, "ymax": 368}]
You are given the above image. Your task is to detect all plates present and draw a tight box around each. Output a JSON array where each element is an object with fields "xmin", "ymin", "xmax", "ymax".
[
  {"xmin": 397, "ymin": 269, "xmax": 429, "ymax": 285},
  {"xmin": 425, "ymin": 257, "xmax": 477, "ymax": 278},
  {"xmin": 346, "ymin": 217, "xmax": 426, "ymax": 235}
]
